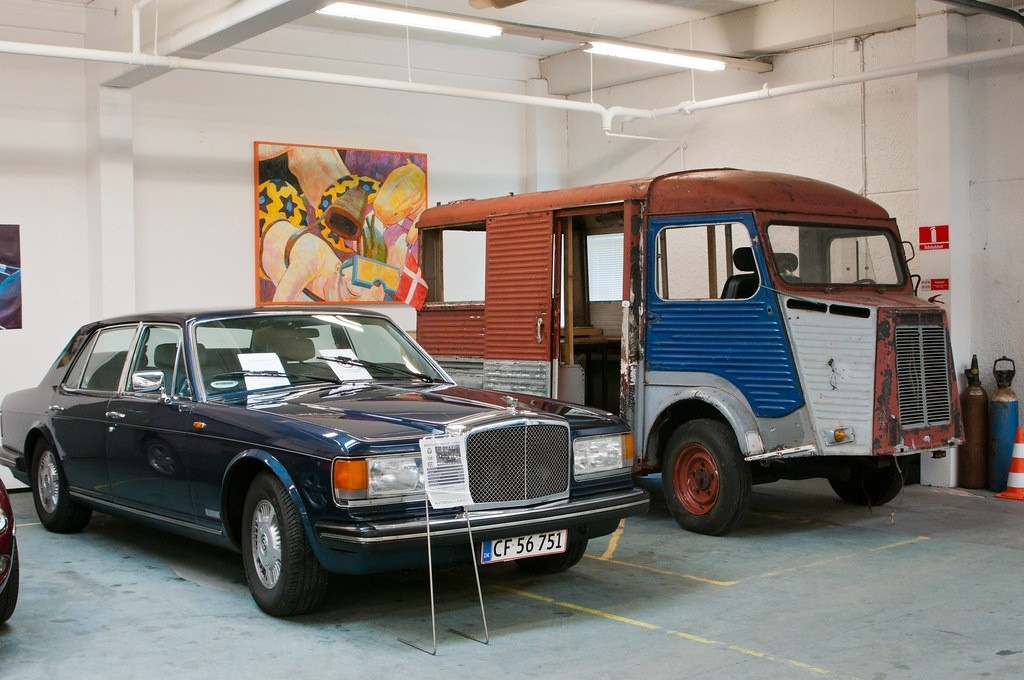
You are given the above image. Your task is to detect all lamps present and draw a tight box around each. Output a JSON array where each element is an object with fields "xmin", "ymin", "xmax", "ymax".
[{"xmin": 313, "ymin": 0, "xmax": 730, "ymax": 73}]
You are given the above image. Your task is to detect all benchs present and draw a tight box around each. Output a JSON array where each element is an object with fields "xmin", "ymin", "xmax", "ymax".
[{"xmin": 75, "ymin": 348, "xmax": 253, "ymax": 395}]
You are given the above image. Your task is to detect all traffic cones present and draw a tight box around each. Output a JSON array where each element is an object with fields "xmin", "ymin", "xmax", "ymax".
[{"xmin": 994, "ymin": 425, "xmax": 1024, "ymax": 502}]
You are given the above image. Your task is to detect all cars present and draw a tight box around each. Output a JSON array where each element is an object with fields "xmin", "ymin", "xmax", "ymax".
[{"xmin": 0, "ymin": 303, "xmax": 653, "ymax": 618}]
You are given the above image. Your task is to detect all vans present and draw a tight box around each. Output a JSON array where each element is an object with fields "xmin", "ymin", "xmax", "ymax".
[{"xmin": 411, "ymin": 165, "xmax": 966, "ymax": 537}]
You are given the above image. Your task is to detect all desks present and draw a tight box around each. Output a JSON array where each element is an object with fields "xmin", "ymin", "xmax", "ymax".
[{"xmin": 557, "ymin": 336, "xmax": 621, "ymax": 410}]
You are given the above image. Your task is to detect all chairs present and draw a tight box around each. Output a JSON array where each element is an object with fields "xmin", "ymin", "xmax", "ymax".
[
  {"xmin": 269, "ymin": 336, "xmax": 336, "ymax": 387},
  {"xmin": 721, "ymin": 246, "xmax": 760, "ymax": 299},
  {"xmin": 773, "ymin": 254, "xmax": 804, "ymax": 284},
  {"xmin": 152, "ymin": 341, "xmax": 227, "ymax": 401}
]
